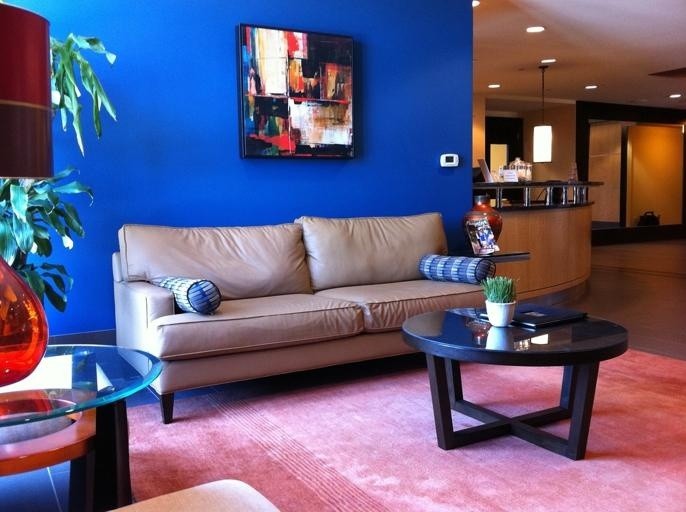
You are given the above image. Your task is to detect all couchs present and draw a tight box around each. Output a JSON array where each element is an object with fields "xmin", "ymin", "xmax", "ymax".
[{"xmin": 111, "ymin": 212, "xmax": 486, "ymax": 424}]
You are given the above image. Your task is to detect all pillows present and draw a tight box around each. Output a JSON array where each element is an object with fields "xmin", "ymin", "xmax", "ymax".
[
  {"xmin": 292, "ymin": 212, "xmax": 449, "ymax": 291},
  {"xmin": 418, "ymin": 253, "xmax": 496, "ymax": 285},
  {"xmin": 118, "ymin": 224, "xmax": 314, "ymax": 301},
  {"xmin": 157, "ymin": 276, "xmax": 222, "ymax": 315}
]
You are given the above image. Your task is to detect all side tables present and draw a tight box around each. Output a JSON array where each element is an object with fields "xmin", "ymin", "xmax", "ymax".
[{"xmin": 451, "ymin": 251, "xmax": 531, "ymax": 263}]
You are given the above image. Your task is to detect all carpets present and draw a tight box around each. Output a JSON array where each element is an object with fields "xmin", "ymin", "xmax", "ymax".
[{"xmin": 127, "ymin": 348, "xmax": 686, "ymax": 512}]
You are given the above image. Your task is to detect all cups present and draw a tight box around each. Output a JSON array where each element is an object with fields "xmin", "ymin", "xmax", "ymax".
[{"xmin": 567, "ymin": 161, "xmax": 578, "ymax": 184}]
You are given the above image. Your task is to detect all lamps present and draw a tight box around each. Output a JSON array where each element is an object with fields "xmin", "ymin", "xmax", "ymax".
[{"xmin": 532, "ymin": 65, "xmax": 553, "ymax": 163}]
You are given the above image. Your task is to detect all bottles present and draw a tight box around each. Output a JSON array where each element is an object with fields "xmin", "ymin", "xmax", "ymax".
[{"xmin": 509, "ymin": 159, "xmax": 532, "ymax": 180}]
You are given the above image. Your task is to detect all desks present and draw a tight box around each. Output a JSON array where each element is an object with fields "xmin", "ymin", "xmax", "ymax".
[{"xmin": 0, "ymin": 343, "xmax": 165, "ymax": 512}]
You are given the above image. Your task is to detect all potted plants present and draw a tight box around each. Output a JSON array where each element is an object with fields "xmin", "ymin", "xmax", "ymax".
[
  {"xmin": 0, "ymin": 167, "xmax": 95, "ymax": 388},
  {"xmin": 479, "ymin": 275, "xmax": 518, "ymax": 328}
]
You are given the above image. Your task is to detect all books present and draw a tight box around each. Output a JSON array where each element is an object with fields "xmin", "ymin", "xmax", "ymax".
[
  {"xmin": 466, "ymin": 217, "xmax": 500, "ymax": 255},
  {"xmin": 478, "ymin": 304, "xmax": 589, "ymax": 328}
]
